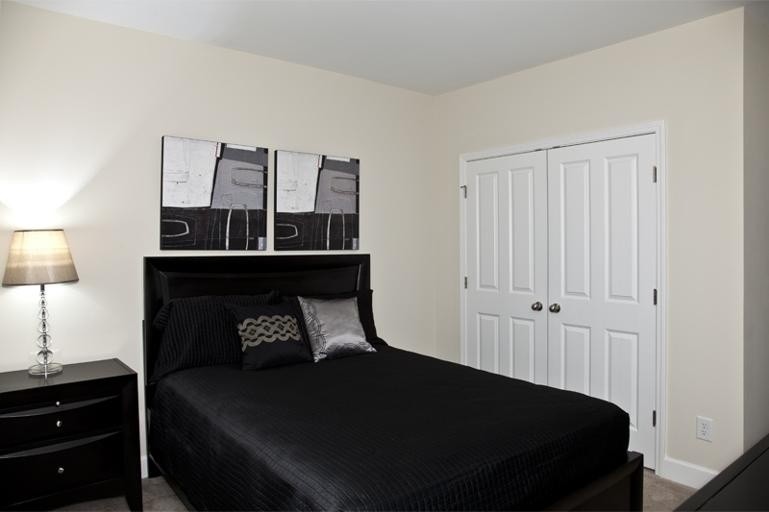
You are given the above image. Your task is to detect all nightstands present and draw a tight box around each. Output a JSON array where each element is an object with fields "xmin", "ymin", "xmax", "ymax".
[{"xmin": 0, "ymin": 358, "xmax": 143, "ymax": 512}]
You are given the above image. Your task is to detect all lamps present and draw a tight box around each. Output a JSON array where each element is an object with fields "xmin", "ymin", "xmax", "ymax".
[{"xmin": 1, "ymin": 229, "xmax": 79, "ymax": 380}]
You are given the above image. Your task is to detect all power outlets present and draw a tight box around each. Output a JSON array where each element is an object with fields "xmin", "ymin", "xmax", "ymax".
[{"xmin": 696, "ymin": 416, "xmax": 712, "ymax": 442}]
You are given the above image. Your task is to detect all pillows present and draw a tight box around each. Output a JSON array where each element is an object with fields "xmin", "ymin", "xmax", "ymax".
[
  {"xmin": 297, "ymin": 295, "xmax": 378, "ymax": 364},
  {"xmin": 225, "ymin": 301, "xmax": 313, "ymax": 370}
]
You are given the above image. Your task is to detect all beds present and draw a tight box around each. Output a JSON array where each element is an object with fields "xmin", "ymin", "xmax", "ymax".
[{"xmin": 143, "ymin": 254, "xmax": 643, "ymax": 511}]
